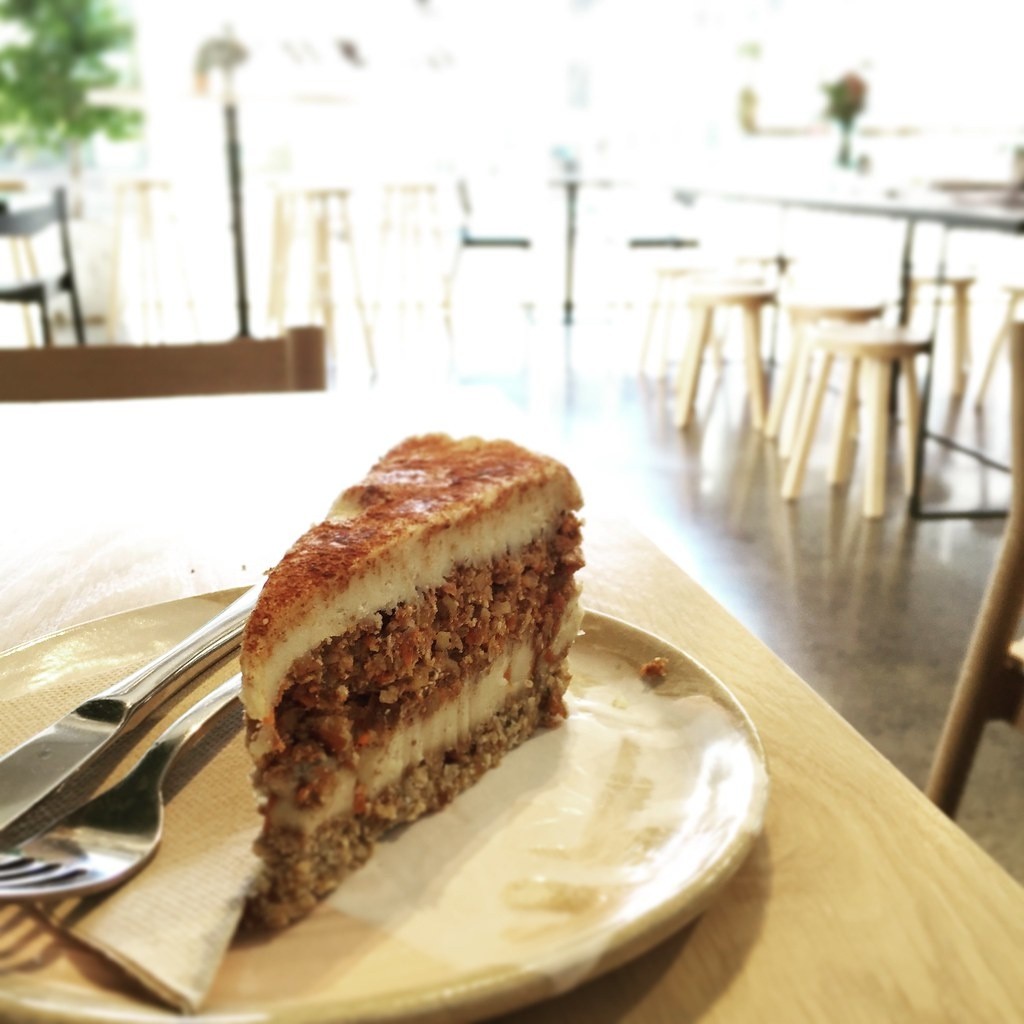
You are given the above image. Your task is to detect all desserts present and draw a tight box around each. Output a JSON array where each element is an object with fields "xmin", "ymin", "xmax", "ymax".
[{"xmin": 241, "ymin": 433, "xmax": 584, "ymax": 925}]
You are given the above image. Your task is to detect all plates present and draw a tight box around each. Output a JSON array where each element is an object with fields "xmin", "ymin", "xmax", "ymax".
[{"xmin": 0, "ymin": 586, "xmax": 771, "ymax": 1024}]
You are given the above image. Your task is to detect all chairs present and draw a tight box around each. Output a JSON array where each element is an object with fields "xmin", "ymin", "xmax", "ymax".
[{"xmin": 0, "ymin": 189, "xmax": 83, "ymax": 347}]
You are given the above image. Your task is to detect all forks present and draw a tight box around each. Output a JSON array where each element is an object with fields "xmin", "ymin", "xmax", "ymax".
[{"xmin": 0, "ymin": 669, "xmax": 242, "ymax": 900}]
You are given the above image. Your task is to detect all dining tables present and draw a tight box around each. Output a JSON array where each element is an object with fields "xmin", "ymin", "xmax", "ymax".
[{"xmin": 0, "ymin": 541, "xmax": 1024, "ymax": 1024}]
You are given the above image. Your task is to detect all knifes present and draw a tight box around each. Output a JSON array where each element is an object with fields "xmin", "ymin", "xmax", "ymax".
[{"xmin": 0, "ymin": 581, "xmax": 263, "ymax": 832}]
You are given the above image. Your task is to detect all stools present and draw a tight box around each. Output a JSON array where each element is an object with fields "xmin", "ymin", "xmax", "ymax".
[{"xmin": 0, "ymin": 172, "xmax": 1024, "ymax": 516}]
